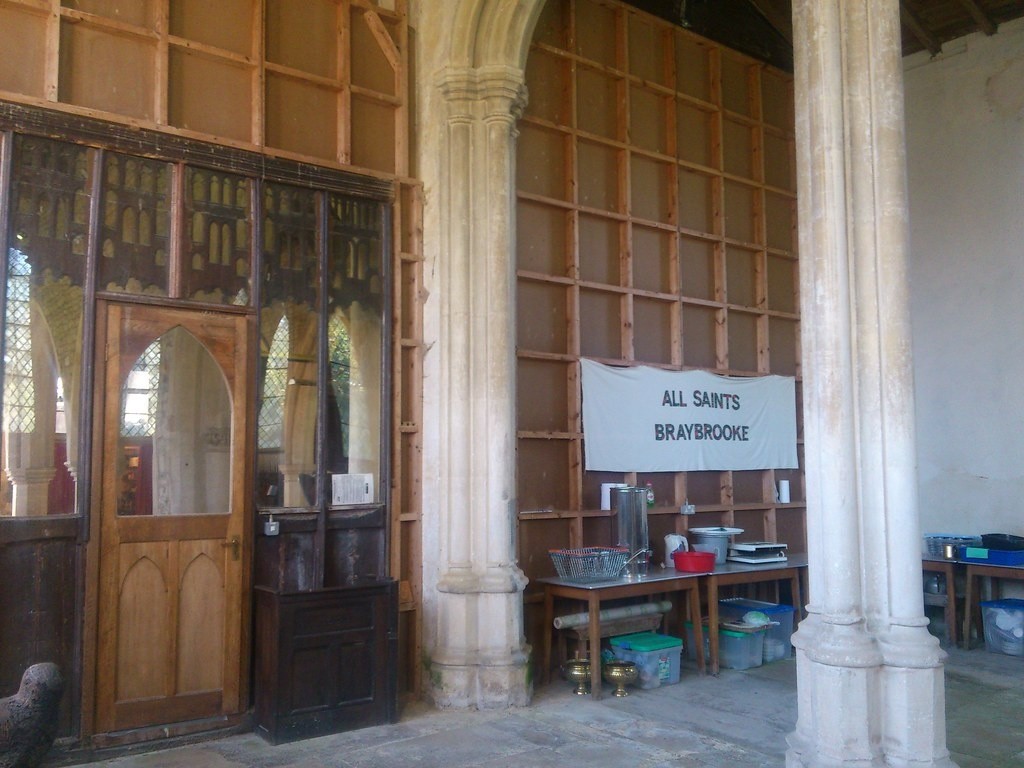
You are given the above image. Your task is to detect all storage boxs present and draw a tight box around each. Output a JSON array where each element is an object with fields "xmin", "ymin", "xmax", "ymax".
[
  {"xmin": 719, "ymin": 598, "xmax": 796, "ymax": 662},
  {"xmin": 684, "ymin": 621, "xmax": 764, "ymax": 670},
  {"xmin": 980, "ymin": 533, "xmax": 1024, "ymax": 551},
  {"xmin": 980, "ymin": 598, "xmax": 1024, "ymax": 658},
  {"xmin": 547, "ymin": 547, "xmax": 628, "ymax": 581},
  {"xmin": 957, "ymin": 546, "xmax": 1024, "ymax": 566},
  {"xmin": 611, "ymin": 633, "xmax": 682, "ymax": 689}
]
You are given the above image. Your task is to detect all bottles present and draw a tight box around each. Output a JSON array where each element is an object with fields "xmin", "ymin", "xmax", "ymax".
[{"xmin": 642, "ymin": 481, "xmax": 655, "ymax": 508}]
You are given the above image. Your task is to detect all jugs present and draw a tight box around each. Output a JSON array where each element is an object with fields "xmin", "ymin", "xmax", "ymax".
[{"xmin": 692, "ymin": 544, "xmax": 720, "ymax": 564}]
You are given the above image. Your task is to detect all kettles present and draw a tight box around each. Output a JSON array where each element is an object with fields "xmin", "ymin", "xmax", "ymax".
[
  {"xmin": 925, "ymin": 572, "xmax": 947, "ymax": 594},
  {"xmin": 663, "ymin": 533, "xmax": 689, "ymax": 568}
]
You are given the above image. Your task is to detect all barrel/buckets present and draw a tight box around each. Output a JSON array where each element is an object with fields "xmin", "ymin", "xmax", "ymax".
[
  {"xmin": 608, "ymin": 487, "xmax": 649, "ymax": 579},
  {"xmin": 693, "ymin": 535, "xmax": 731, "ymax": 564},
  {"xmin": 601, "ymin": 483, "xmax": 627, "ymax": 510}
]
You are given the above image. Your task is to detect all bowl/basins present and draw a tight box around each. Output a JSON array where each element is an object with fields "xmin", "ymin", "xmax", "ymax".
[{"xmin": 674, "ymin": 551, "xmax": 717, "ymax": 573}]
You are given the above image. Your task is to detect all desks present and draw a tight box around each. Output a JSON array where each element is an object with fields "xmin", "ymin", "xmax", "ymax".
[
  {"xmin": 922, "ymin": 554, "xmax": 958, "ymax": 643},
  {"xmin": 536, "ymin": 567, "xmax": 707, "ymax": 698},
  {"xmin": 707, "ymin": 563, "xmax": 808, "ymax": 675},
  {"xmin": 958, "ymin": 561, "xmax": 1024, "ymax": 652}
]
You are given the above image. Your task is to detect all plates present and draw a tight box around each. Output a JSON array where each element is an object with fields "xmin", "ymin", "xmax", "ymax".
[
  {"xmin": 757, "ymin": 639, "xmax": 784, "ymax": 658},
  {"xmin": 688, "ymin": 528, "xmax": 745, "ymax": 535},
  {"xmin": 1002, "ymin": 641, "xmax": 1023, "ymax": 655}
]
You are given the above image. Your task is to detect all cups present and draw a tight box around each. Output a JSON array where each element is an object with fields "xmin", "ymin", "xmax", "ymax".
[
  {"xmin": 945, "ymin": 544, "xmax": 957, "ymax": 559},
  {"xmin": 778, "ymin": 479, "xmax": 791, "ymax": 504}
]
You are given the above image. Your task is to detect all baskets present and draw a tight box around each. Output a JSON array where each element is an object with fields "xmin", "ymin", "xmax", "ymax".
[{"xmin": 549, "ymin": 546, "xmax": 629, "ymax": 584}]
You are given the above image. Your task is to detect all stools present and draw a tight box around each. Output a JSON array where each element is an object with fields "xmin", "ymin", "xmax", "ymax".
[{"xmin": 559, "ymin": 614, "xmax": 662, "ymax": 680}]
